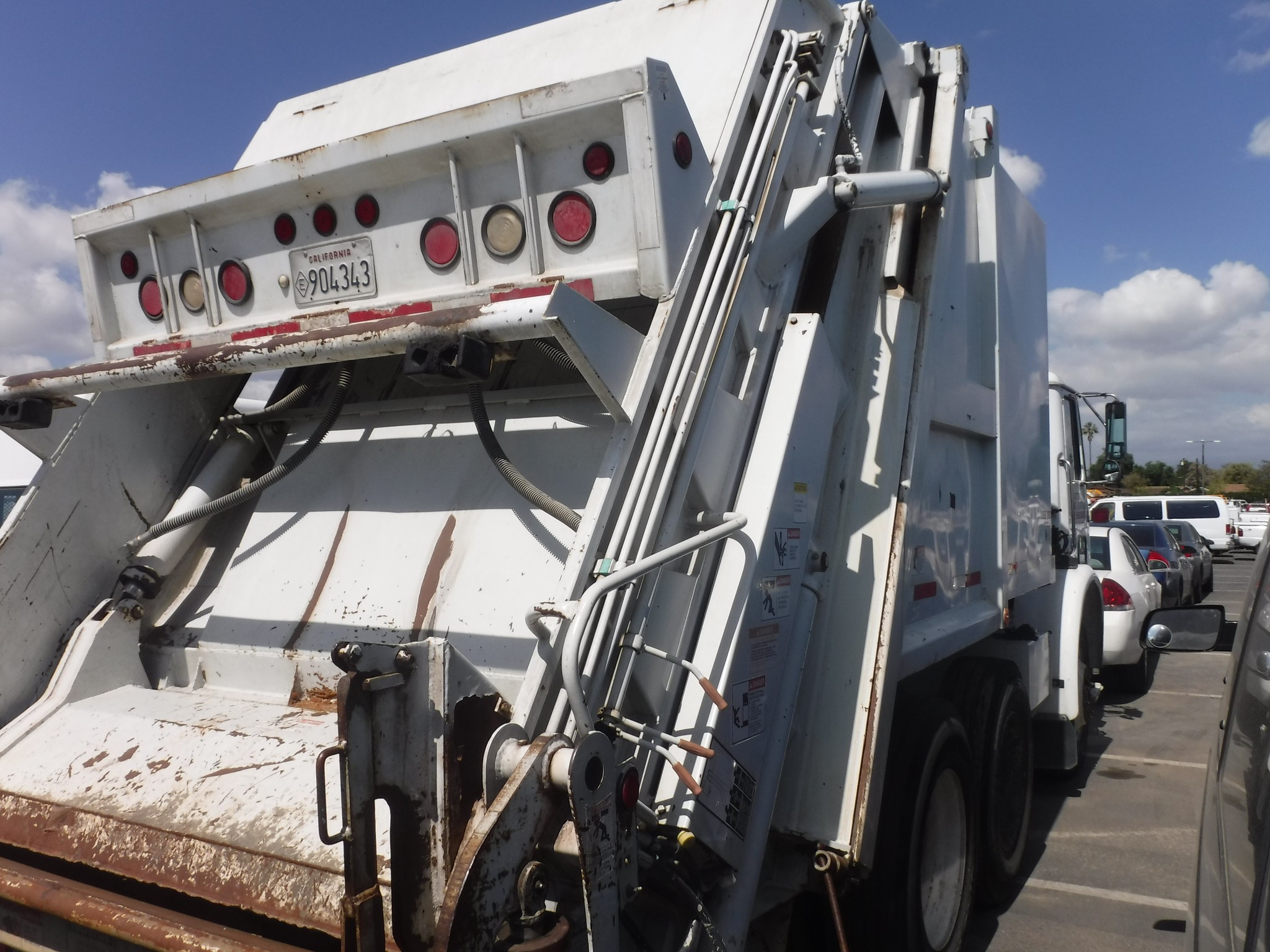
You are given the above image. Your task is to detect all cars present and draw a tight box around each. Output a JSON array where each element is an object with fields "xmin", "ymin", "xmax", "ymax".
[
  {"xmin": 1147, "ymin": 520, "xmax": 1215, "ymax": 604},
  {"xmin": 1086, "ymin": 526, "xmax": 1169, "ymax": 694},
  {"xmin": 1212, "ymin": 495, "xmax": 1270, "ymax": 555},
  {"xmin": 1090, "ymin": 519, "xmax": 1198, "ymax": 607}
]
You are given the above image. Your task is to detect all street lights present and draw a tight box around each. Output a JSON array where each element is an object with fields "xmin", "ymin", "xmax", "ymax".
[{"xmin": 1185, "ymin": 439, "xmax": 1221, "ymax": 495}]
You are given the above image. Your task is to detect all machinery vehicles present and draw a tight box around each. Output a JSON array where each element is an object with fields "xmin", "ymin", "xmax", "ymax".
[{"xmin": 0, "ymin": 0, "xmax": 1127, "ymax": 952}]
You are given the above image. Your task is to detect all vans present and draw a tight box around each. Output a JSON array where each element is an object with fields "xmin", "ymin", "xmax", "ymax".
[
  {"xmin": 1139, "ymin": 517, "xmax": 1270, "ymax": 952},
  {"xmin": 1088, "ymin": 495, "xmax": 1236, "ymax": 556}
]
